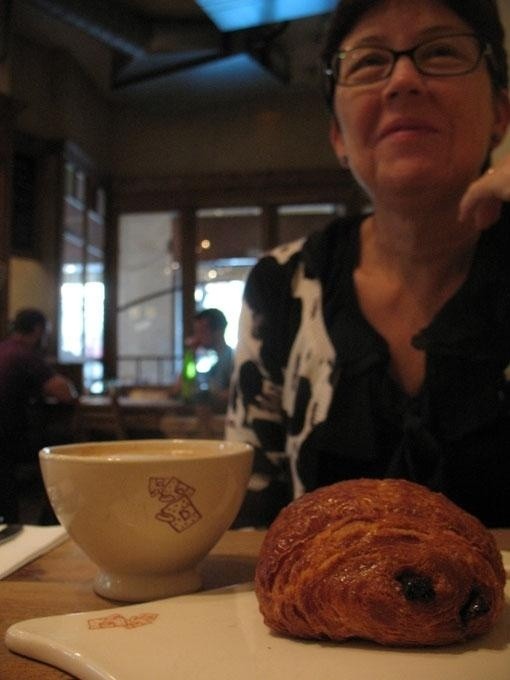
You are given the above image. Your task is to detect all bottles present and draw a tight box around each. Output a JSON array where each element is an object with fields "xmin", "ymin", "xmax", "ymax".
[{"xmin": 182, "ymin": 346, "xmax": 196, "ymax": 398}]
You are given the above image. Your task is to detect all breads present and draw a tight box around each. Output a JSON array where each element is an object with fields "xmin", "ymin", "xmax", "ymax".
[{"xmin": 253, "ymin": 479, "xmax": 507, "ymax": 648}]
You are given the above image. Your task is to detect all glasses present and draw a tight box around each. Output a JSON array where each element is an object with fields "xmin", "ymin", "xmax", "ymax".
[{"xmin": 327, "ymin": 33, "xmax": 487, "ymax": 87}]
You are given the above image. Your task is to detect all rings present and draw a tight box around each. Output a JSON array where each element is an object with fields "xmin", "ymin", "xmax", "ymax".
[{"xmin": 486, "ymin": 166, "xmax": 496, "ymax": 176}]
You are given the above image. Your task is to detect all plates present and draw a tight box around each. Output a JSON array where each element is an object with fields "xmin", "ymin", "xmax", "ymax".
[{"xmin": 6, "ymin": 546, "xmax": 510, "ymax": 679}]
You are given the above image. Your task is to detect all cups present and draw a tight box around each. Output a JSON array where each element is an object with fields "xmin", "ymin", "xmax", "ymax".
[{"xmin": 40, "ymin": 437, "xmax": 254, "ymax": 603}]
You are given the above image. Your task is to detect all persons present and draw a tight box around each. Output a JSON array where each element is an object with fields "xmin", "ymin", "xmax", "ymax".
[
  {"xmin": 167, "ymin": 309, "xmax": 236, "ymax": 414},
  {"xmin": 223, "ymin": 0, "xmax": 510, "ymax": 531},
  {"xmin": 0, "ymin": 309, "xmax": 79, "ymax": 407}
]
